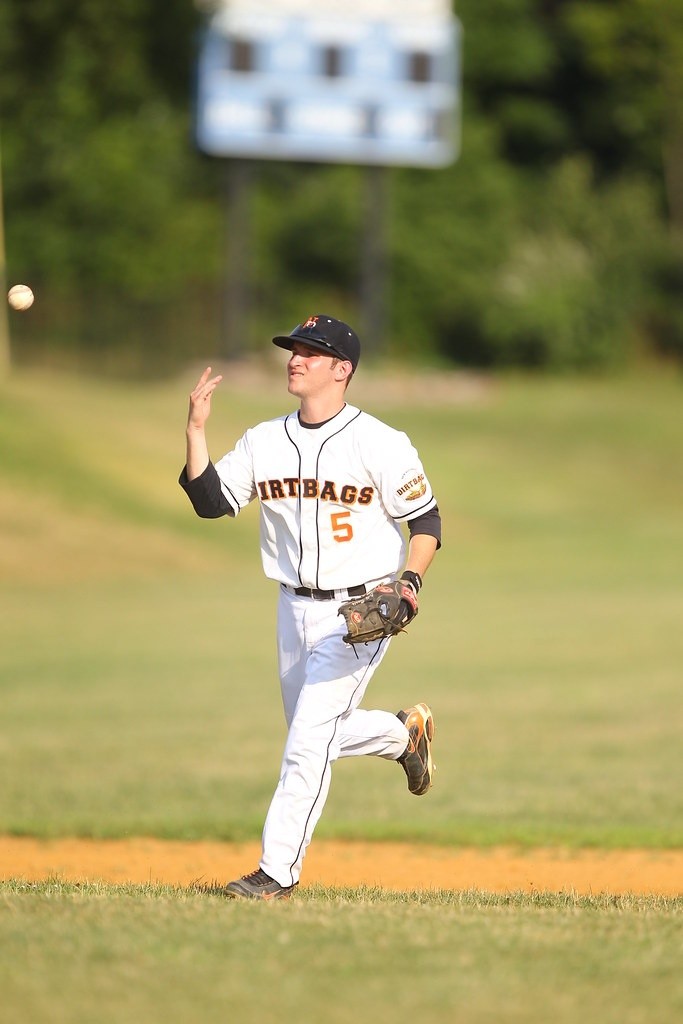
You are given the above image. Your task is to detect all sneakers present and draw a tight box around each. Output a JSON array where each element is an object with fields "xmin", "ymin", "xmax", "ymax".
[
  {"xmin": 396, "ymin": 703, "xmax": 435, "ymax": 796},
  {"xmin": 225, "ymin": 868, "xmax": 300, "ymax": 902}
]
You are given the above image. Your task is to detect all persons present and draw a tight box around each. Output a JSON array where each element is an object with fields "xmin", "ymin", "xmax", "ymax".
[{"xmin": 179, "ymin": 316, "xmax": 445, "ymax": 901}]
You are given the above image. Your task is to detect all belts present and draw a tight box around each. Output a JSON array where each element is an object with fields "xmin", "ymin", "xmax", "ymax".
[{"xmin": 281, "ymin": 581, "xmax": 366, "ymax": 600}]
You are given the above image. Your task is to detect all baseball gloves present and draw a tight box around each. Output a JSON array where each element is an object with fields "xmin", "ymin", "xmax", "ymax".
[{"xmin": 336, "ymin": 580, "xmax": 420, "ymax": 645}]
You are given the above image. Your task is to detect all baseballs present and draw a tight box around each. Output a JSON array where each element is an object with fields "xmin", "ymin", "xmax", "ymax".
[{"xmin": 7, "ymin": 283, "xmax": 35, "ymax": 313}]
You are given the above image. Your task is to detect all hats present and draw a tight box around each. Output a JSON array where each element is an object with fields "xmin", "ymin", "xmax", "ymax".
[{"xmin": 272, "ymin": 314, "xmax": 362, "ymax": 375}]
